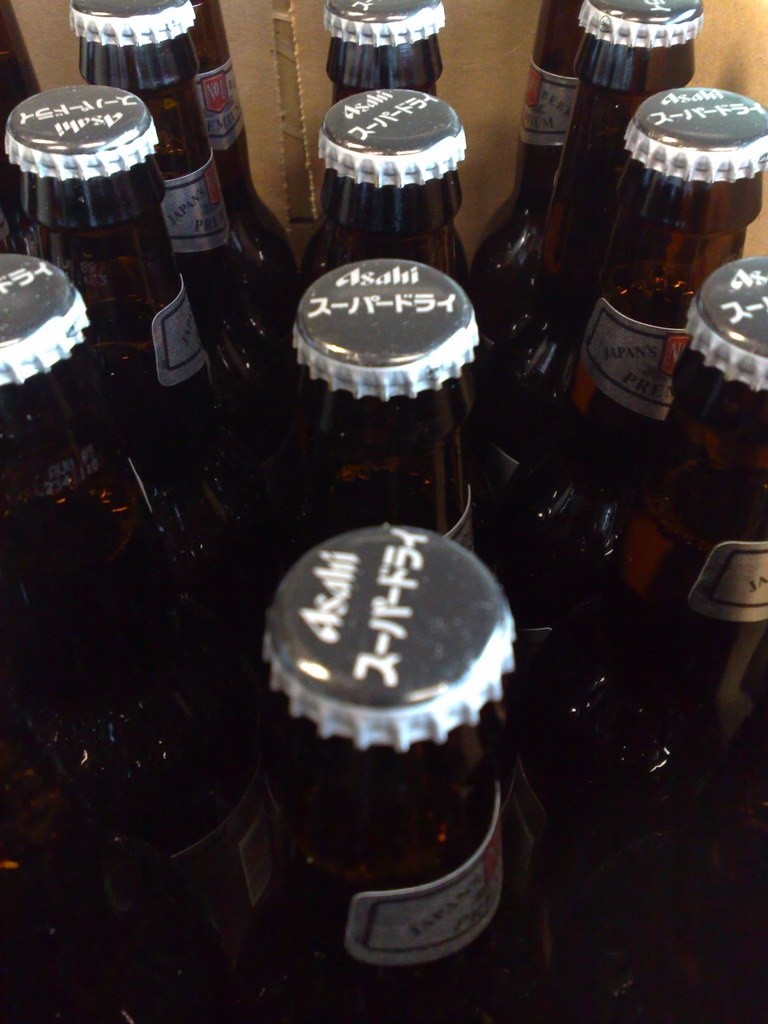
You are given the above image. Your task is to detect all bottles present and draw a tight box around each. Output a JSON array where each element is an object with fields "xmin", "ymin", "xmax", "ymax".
[
  {"xmin": 471, "ymin": 0, "xmax": 768, "ymax": 1024},
  {"xmin": 259, "ymin": 0, "xmax": 518, "ymax": 1024},
  {"xmin": 0, "ymin": 0, "xmax": 286, "ymax": 1024}
]
